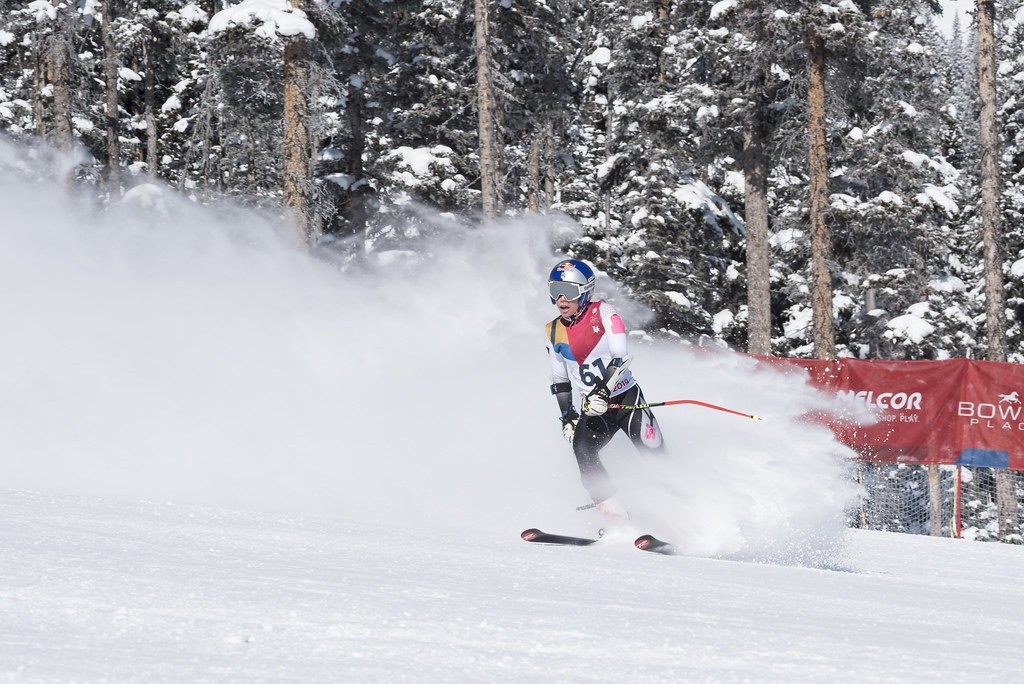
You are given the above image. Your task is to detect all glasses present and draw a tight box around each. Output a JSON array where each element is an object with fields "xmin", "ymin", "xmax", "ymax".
[{"xmin": 547, "ymin": 281, "xmax": 582, "ymax": 302}]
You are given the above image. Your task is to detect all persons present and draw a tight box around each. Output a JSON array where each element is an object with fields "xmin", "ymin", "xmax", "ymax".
[{"xmin": 545, "ymin": 260, "xmax": 677, "ymax": 522}]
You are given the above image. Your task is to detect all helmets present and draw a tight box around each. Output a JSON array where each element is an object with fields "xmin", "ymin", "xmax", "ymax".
[{"xmin": 549, "ymin": 259, "xmax": 596, "ymax": 308}]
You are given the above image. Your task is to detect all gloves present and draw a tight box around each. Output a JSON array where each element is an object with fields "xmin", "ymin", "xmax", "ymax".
[
  {"xmin": 561, "ymin": 420, "xmax": 577, "ymax": 443},
  {"xmin": 581, "ymin": 394, "xmax": 609, "ymax": 416}
]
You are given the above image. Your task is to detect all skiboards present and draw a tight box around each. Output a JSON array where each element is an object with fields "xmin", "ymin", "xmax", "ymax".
[{"xmin": 520, "ymin": 527, "xmax": 850, "ymax": 576}]
